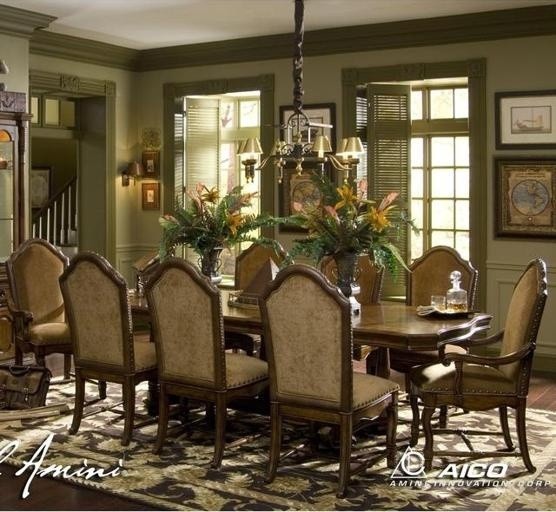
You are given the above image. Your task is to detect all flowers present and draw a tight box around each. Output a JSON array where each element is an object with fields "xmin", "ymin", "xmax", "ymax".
[
  {"xmin": 279, "ymin": 178, "xmax": 419, "ymax": 282},
  {"xmin": 157, "ymin": 184, "xmax": 280, "ymax": 264}
]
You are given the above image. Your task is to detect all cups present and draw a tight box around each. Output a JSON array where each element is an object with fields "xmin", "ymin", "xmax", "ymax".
[{"xmin": 431, "ymin": 295, "xmax": 445, "ymax": 311}]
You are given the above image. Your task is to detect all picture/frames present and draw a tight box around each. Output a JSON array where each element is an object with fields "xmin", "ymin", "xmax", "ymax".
[
  {"xmin": 277, "ymin": 102, "xmax": 337, "ymax": 157},
  {"xmin": 142, "ymin": 150, "xmax": 159, "ymax": 179},
  {"xmin": 492, "ymin": 88, "xmax": 555, "ymax": 151},
  {"xmin": 272, "ymin": 163, "xmax": 339, "ymax": 235},
  {"xmin": 142, "ymin": 183, "xmax": 160, "ymax": 213},
  {"xmin": 492, "ymin": 156, "xmax": 556, "ymax": 243}
]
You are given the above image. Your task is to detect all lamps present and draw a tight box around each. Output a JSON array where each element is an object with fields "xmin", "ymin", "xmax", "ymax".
[
  {"xmin": 237, "ymin": 0, "xmax": 364, "ymax": 185},
  {"xmin": 121, "ymin": 160, "xmax": 139, "ymax": 190}
]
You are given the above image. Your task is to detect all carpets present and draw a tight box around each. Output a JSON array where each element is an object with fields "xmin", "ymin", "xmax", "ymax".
[{"xmin": 0, "ymin": 368, "xmax": 555, "ymax": 512}]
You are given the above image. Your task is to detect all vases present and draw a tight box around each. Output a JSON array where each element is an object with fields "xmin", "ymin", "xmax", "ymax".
[
  {"xmin": 196, "ymin": 247, "xmax": 223, "ymax": 290},
  {"xmin": 330, "ymin": 253, "xmax": 362, "ymax": 328}
]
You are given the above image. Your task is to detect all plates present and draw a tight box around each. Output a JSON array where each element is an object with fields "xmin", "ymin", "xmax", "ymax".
[{"xmin": 417, "ymin": 310, "xmax": 474, "ymax": 318}]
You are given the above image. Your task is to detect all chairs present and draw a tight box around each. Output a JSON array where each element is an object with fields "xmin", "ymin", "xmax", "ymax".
[
  {"xmin": 419, "ymin": 255, "xmax": 550, "ymax": 472},
  {"xmin": 57, "ymin": 250, "xmax": 161, "ymax": 449},
  {"xmin": 2, "ymin": 237, "xmax": 110, "ymax": 398},
  {"xmin": 143, "ymin": 258, "xmax": 270, "ymax": 470},
  {"xmin": 226, "ymin": 239, "xmax": 297, "ymax": 356},
  {"xmin": 310, "ymin": 251, "xmax": 384, "ymax": 398},
  {"xmin": 364, "ymin": 245, "xmax": 478, "ymax": 428},
  {"xmin": 258, "ymin": 263, "xmax": 404, "ymax": 498}
]
